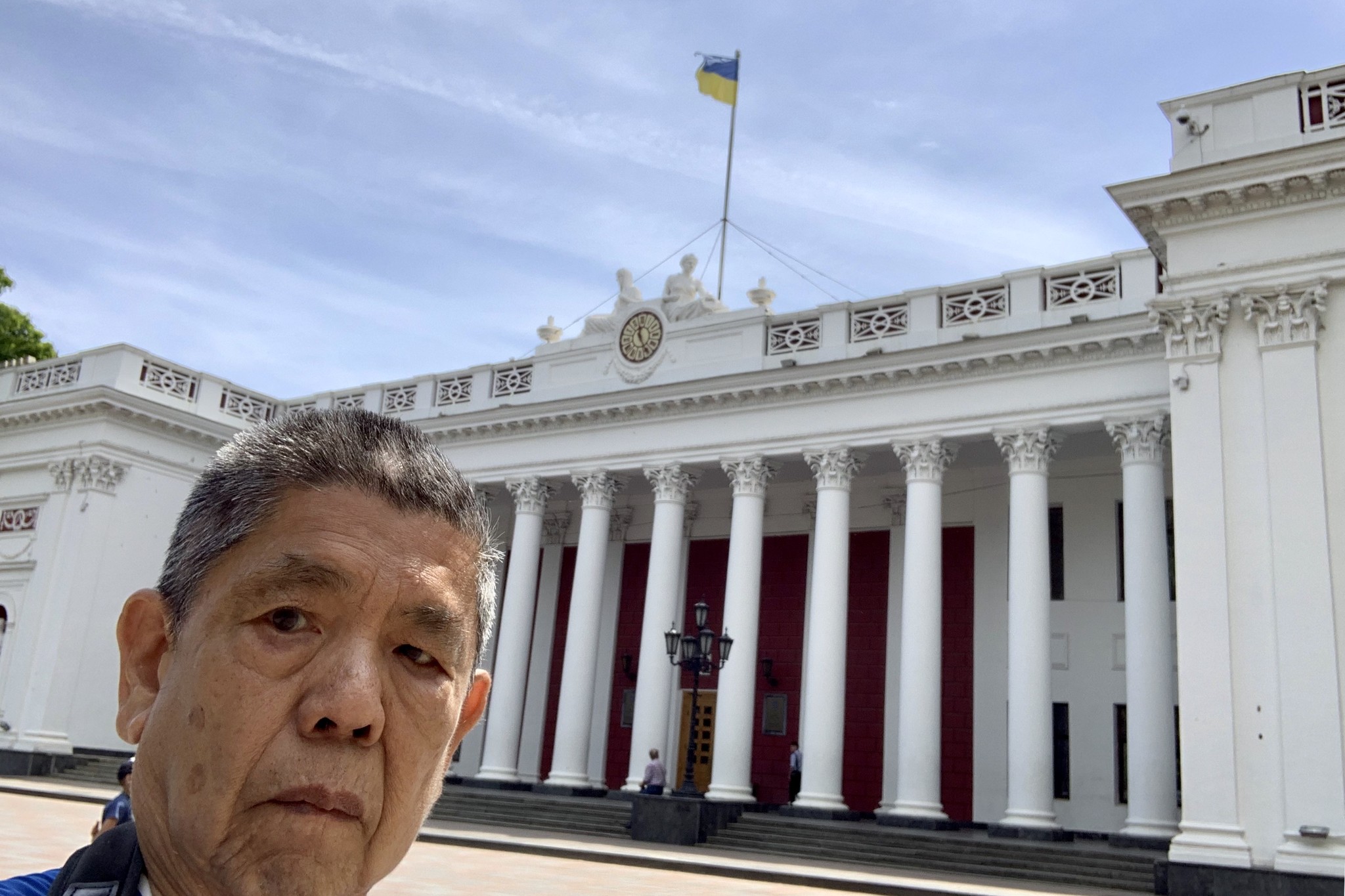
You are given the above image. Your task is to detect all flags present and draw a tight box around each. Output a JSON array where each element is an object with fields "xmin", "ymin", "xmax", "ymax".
[{"xmin": 694, "ymin": 51, "xmax": 735, "ymax": 106}]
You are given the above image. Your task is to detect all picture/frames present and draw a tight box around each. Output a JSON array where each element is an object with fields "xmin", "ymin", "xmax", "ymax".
[
  {"xmin": 761, "ymin": 693, "xmax": 788, "ymax": 736},
  {"xmin": 621, "ymin": 689, "xmax": 635, "ymax": 728}
]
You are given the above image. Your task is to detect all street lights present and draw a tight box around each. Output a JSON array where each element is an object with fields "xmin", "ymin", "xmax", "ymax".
[{"xmin": 663, "ymin": 596, "xmax": 735, "ymax": 799}]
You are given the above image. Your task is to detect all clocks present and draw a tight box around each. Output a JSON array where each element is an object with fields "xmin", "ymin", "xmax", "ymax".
[{"xmin": 617, "ymin": 306, "xmax": 668, "ymax": 367}]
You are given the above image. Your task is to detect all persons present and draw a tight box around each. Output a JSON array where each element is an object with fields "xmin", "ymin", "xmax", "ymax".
[
  {"xmin": 0, "ymin": 408, "xmax": 503, "ymax": 896},
  {"xmin": 576, "ymin": 268, "xmax": 642, "ymax": 335},
  {"xmin": 623, "ymin": 748, "xmax": 667, "ymax": 829},
  {"xmin": 661, "ymin": 253, "xmax": 715, "ymax": 324},
  {"xmin": 787, "ymin": 742, "xmax": 802, "ymax": 805},
  {"xmin": 90, "ymin": 760, "xmax": 134, "ymax": 842}
]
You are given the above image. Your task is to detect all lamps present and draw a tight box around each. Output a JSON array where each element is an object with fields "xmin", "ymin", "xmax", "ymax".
[
  {"xmin": 620, "ymin": 650, "xmax": 636, "ymax": 679},
  {"xmin": 1070, "ymin": 312, "xmax": 1090, "ymax": 324},
  {"xmin": 1299, "ymin": 824, "xmax": 1330, "ymax": 841},
  {"xmin": 781, "ymin": 358, "xmax": 797, "ymax": 367},
  {"xmin": 760, "ymin": 652, "xmax": 774, "ymax": 685}
]
